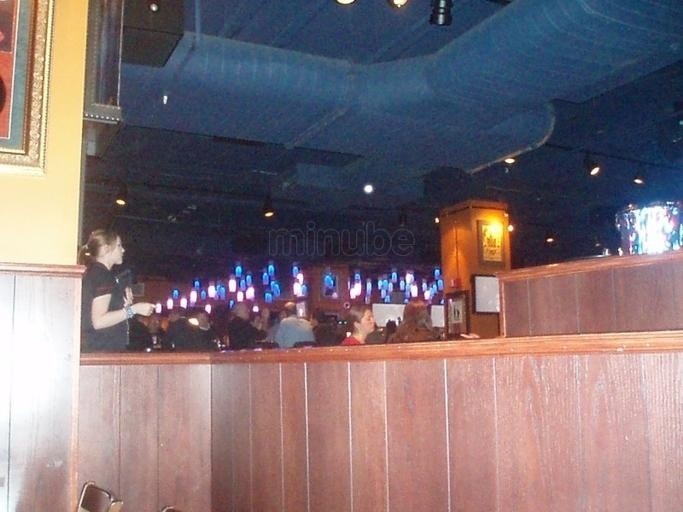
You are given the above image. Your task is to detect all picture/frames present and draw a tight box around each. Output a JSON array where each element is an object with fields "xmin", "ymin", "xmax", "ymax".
[
  {"xmin": 445, "ymin": 291, "xmax": 468, "ymax": 335},
  {"xmin": 0, "ymin": 0, "xmax": 55, "ymax": 176}
]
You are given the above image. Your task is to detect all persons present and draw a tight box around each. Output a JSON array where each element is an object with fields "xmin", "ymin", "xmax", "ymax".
[{"xmin": 76, "ymin": 227, "xmax": 157, "ymax": 354}]
